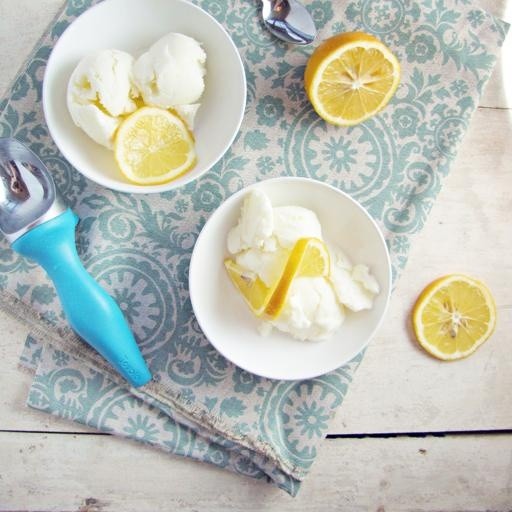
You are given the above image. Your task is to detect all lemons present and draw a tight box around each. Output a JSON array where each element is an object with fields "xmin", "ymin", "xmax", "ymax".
[
  {"xmin": 412, "ymin": 275, "xmax": 496, "ymax": 360},
  {"xmin": 112, "ymin": 107, "xmax": 196, "ymax": 185},
  {"xmin": 225, "ymin": 237, "xmax": 331, "ymax": 322},
  {"xmin": 304, "ymin": 32, "xmax": 400, "ymax": 124}
]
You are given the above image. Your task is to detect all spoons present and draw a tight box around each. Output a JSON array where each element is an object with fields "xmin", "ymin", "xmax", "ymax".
[
  {"xmin": 0, "ymin": 139, "xmax": 154, "ymax": 386},
  {"xmin": 257, "ymin": 0, "xmax": 318, "ymax": 47}
]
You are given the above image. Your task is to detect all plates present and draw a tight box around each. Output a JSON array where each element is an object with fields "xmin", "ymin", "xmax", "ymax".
[
  {"xmin": 187, "ymin": 177, "xmax": 394, "ymax": 386},
  {"xmin": 41, "ymin": 0, "xmax": 248, "ymax": 196}
]
